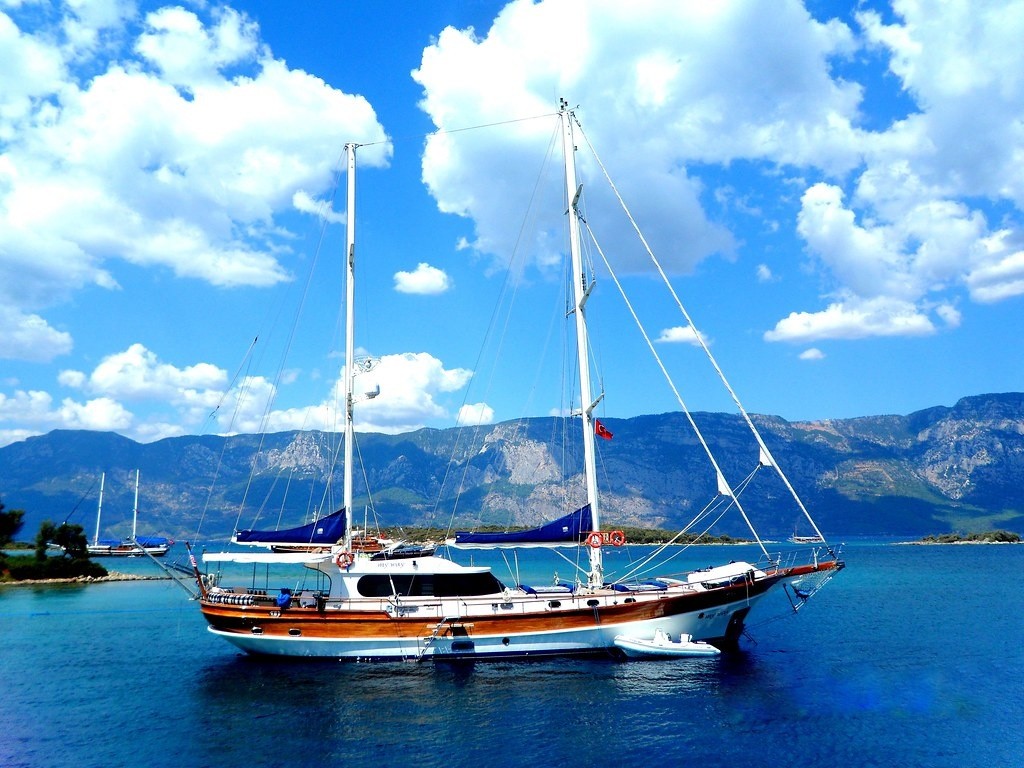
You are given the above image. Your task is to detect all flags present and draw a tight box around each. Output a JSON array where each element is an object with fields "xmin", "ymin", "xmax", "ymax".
[{"xmin": 594, "ymin": 417, "xmax": 613, "ymax": 441}]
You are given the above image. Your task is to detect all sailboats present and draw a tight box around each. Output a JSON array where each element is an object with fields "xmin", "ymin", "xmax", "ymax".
[
  {"xmin": 45, "ymin": 468, "xmax": 176, "ymax": 556},
  {"xmin": 129, "ymin": 93, "xmax": 849, "ymax": 665}
]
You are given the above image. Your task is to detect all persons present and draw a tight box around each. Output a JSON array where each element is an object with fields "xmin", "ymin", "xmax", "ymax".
[{"xmin": 381, "ymin": 534, "xmax": 385, "ymax": 539}]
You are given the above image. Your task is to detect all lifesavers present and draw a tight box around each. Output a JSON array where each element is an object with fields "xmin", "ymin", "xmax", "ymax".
[
  {"xmin": 588, "ymin": 533, "xmax": 603, "ymax": 548},
  {"xmin": 609, "ymin": 531, "xmax": 625, "ymax": 546},
  {"xmin": 336, "ymin": 553, "xmax": 354, "ymax": 568}
]
